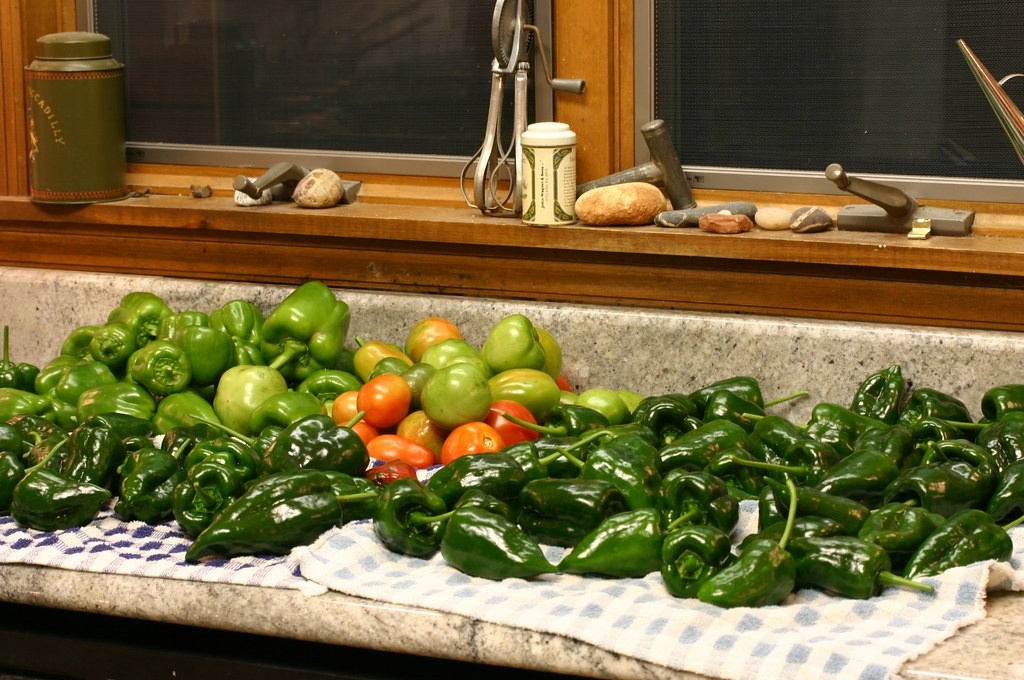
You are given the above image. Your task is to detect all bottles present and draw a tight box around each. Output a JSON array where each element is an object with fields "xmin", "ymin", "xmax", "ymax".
[
  {"xmin": 25, "ymin": 32, "xmax": 130, "ymax": 206},
  {"xmin": 522, "ymin": 120, "xmax": 578, "ymax": 228}
]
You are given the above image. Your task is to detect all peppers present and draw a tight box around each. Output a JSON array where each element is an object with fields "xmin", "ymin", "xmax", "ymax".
[
  {"xmin": 374, "ymin": 365, "xmax": 1024, "ymax": 608},
  {"xmin": 0, "ymin": 281, "xmax": 376, "ymax": 560}
]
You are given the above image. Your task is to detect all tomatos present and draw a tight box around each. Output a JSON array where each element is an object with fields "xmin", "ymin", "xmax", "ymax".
[{"xmin": 331, "ymin": 314, "xmax": 643, "ymax": 472}]
